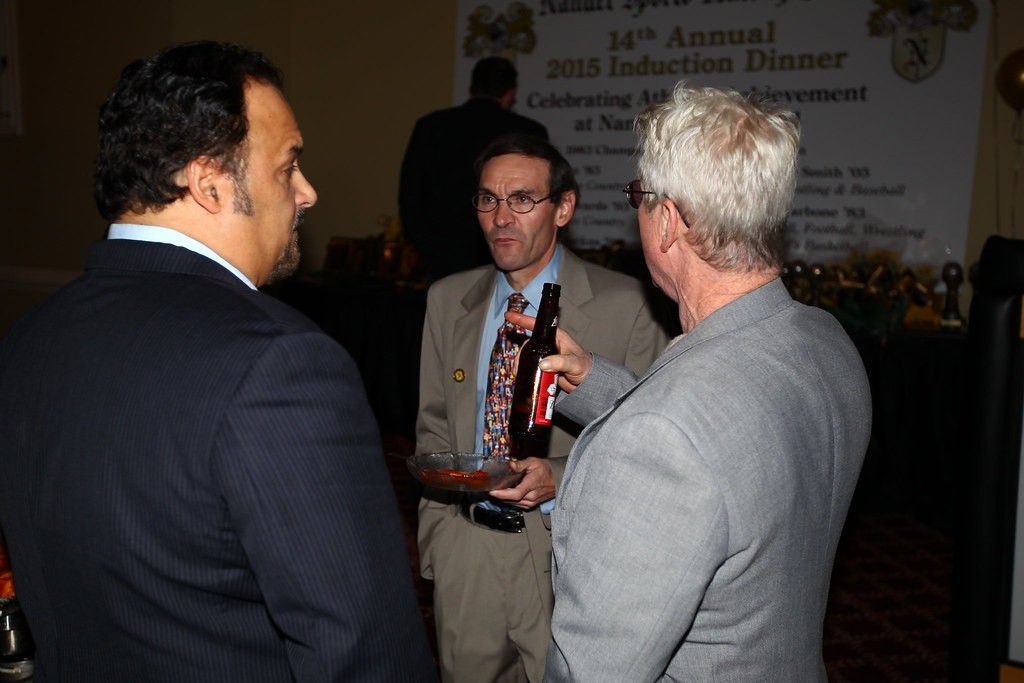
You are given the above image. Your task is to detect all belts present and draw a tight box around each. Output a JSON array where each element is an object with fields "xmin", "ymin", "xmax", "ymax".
[{"xmin": 457, "ymin": 502, "xmax": 526, "ymax": 534}]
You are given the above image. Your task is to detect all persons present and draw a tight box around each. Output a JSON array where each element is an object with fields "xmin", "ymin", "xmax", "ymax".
[
  {"xmin": 505, "ymin": 86, "xmax": 872, "ymax": 683},
  {"xmin": 414, "ymin": 138, "xmax": 671, "ymax": 683},
  {"xmin": 0, "ymin": 42, "xmax": 439, "ymax": 683},
  {"xmin": 398, "ymin": 56, "xmax": 551, "ymax": 409}
]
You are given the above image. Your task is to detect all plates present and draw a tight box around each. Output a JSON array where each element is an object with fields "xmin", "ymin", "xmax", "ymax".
[{"xmin": 407, "ymin": 453, "xmax": 525, "ymax": 491}]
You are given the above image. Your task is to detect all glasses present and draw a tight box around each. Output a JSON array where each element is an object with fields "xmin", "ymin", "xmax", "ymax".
[
  {"xmin": 622, "ymin": 185, "xmax": 698, "ymax": 232},
  {"xmin": 469, "ymin": 194, "xmax": 560, "ymax": 213}
]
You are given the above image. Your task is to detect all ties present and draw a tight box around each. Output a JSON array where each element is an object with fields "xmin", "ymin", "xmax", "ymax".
[{"xmin": 486, "ymin": 294, "xmax": 532, "ymax": 466}]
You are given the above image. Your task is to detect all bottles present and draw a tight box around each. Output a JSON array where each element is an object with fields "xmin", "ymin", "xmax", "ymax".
[{"xmin": 509, "ymin": 283, "xmax": 564, "ymax": 458}]
